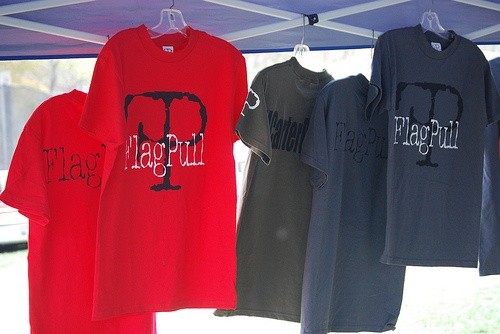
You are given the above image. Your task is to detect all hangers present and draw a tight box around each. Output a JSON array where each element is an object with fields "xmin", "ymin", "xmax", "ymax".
[
  {"xmin": 146, "ymin": 1, "xmax": 187, "ymax": 39},
  {"xmin": 421, "ymin": 0, "xmax": 449, "ymax": 39},
  {"xmin": 293, "ymin": 15, "xmax": 324, "ymax": 72}
]
